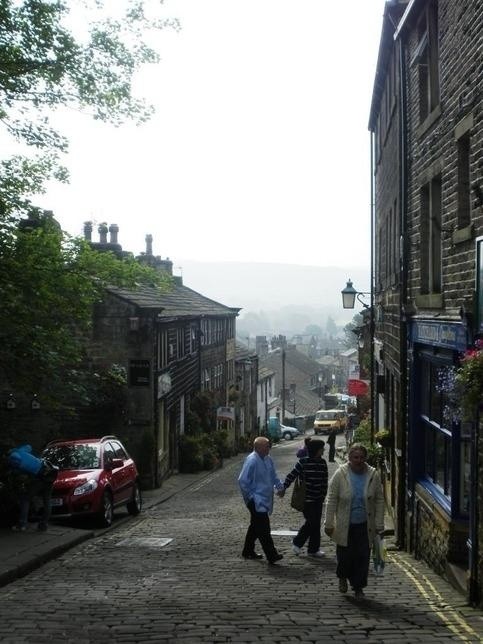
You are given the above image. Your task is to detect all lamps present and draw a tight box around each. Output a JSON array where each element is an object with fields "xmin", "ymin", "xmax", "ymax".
[
  {"xmin": 7, "ymin": 394, "xmax": 16, "ymax": 410},
  {"xmin": 31, "ymin": 394, "xmax": 41, "ymax": 409},
  {"xmin": 356, "ymin": 326, "xmax": 369, "ymax": 348},
  {"xmin": 340, "ymin": 278, "xmax": 371, "ymax": 312},
  {"xmin": 192, "ymin": 325, "xmax": 204, "ymax": 337}
]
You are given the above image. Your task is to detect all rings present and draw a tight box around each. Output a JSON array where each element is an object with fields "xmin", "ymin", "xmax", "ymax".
[{"xmin": 328, "ymin": 530, "xmax": 331, "ymax": 533}]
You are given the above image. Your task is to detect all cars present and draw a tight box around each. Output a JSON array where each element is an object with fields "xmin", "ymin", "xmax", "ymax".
[
  {"xmin": 340, "ymin": 395, "xmax": 353, "ymax": 409},
  {"xmin": 267, "ymin": 421, "xmax": 299, "ymax": 441}
]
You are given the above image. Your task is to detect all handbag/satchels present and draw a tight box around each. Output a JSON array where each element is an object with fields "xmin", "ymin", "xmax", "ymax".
[
  {"xmin": 373, "ymin": 535, "xmax": 385, "ymax": 575},
  {"xmin": 44, "ymin": 461, "xmax": 59, "ymax": 484},
  {"xmin": 291, "ymin": 483, "xmax": 306, "ymax": 512}
]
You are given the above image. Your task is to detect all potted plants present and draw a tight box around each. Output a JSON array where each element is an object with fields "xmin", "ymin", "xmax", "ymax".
[{"xmin": 374, "ymin": 430, "xmax": 392, "ymax": 447}]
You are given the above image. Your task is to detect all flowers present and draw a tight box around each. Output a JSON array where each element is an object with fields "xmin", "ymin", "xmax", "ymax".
[{"xmin": 435, "ymin": 338, "xmax": 483, "ymax": 425}]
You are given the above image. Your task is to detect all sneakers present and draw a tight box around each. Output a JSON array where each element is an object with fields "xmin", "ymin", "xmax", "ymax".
[
  {"xmin": 338, "ymin": 578, "xmax": 348, "ymax": 593},
  {"xmin": 34, "ymin": 524, "xmax": 46, "ymax": 531},
  {"xmin": 244, "ymin": 541, "xmax": 325, "ymax": 564},
  {"xmin": 356, "ymin": 593, "xmax": 364, "ymax": 604},
  {"xmin": 12, "ymin": 525, "xmax": 25, "ymax": 531}
]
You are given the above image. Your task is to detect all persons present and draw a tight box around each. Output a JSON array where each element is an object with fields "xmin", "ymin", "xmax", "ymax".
[
  {"xmin": 237, "ymin": 437, "xmax": 284, "ymax": 564},
  {"xmin": 0, "ymin": 444, "xmax": 53, "ymax": 531},
  {"xmin": 325, "ymin": 426, "xmax": 337, "ymax": 462},
  {"xmin": 297, "ymin": 437, "xmax": 312, "ymax": 458},
  {"xmin": 277, "ymin": 439, "xmax": 327, "ymax": 558},
  {"xmin": 324, "ymin": 445, "xmax": 384, "ymax": 594}
]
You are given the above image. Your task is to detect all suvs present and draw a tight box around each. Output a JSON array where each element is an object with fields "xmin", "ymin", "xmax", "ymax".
[
  {"xmin": 313, "ymin": 409, "xmax": 348, "ymax": 434},
  {"xmin": 35, "ymin": 435, "xmax": 142, "ymax": 528}
]
